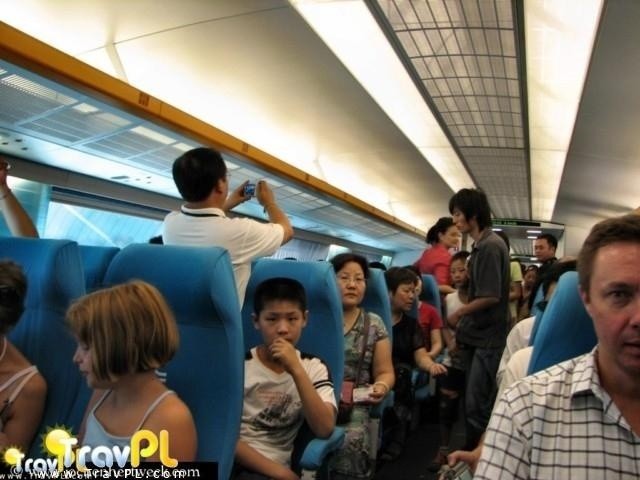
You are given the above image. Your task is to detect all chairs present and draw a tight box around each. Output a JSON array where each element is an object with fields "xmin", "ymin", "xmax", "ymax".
[{"xmin": 529, "ymin": 271, "xmax": 598, "ymax": 382}]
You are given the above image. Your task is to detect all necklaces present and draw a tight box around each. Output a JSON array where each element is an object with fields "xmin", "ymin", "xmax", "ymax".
[{"xmin": 0, "ymin": 335, "xmax": 8, "ymax": 361}]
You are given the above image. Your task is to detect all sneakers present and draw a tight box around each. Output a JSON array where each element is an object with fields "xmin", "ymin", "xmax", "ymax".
[{"xmin": 427, "ymin": 452, "xmax": 448, "ymax": 473}]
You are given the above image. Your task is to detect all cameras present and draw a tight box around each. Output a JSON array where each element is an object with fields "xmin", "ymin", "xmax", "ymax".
[{"xmin": 243, "ymin": 184, "xmax": 256, "ymax": 197}]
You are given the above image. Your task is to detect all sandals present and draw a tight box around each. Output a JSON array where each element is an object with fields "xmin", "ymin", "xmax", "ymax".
[{"xmin": 382, "ymin": 440, "xmax": 404, "ymax": 462}]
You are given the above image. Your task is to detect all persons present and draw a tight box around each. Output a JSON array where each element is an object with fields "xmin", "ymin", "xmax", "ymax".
[
  {"xmin": 60, "ymin": 277, "xmax": 197, "ymax": 464},
  {"xmin": 160, "ymin": 147, "xmax": 295, "ymax": 314},
  {"xmin": 0, "ymin": 257, "xmax": 48, "ymax": 466},
  {"xmin": 474, "ymin": 212, "xmax": 640, "ymax": 480},
  {"xmin": 415, "ymin": 213, "xmax": 463, "ymax": 293},
  {"xmin": 142, "ymin": 230, "xmax": 467, "ymax": 479},
  {"xmin": 444, "ymin": 254, "xmax": 581, "ymax": 480},
  {"xmin": 0, "ymin": 154, "xmax": 40, "ymax": 241},
  {"xmin": 492, "ymin": 226, "xmax": 587, "ymax": 410},
  {"xmin": 451, "ymin": 187, "xmax": 510, "ymax": 451}
]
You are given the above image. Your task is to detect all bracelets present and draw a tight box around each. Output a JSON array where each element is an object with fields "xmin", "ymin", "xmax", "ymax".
[{"xmin": 2, "ymin": 187, "xmax": 12, "ymax": 201}]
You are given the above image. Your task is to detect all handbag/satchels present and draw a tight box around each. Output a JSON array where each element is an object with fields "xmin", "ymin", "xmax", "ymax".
[{"xmin": 336, "ymin": 380, "xmax": 357, "ymax": 422}]
